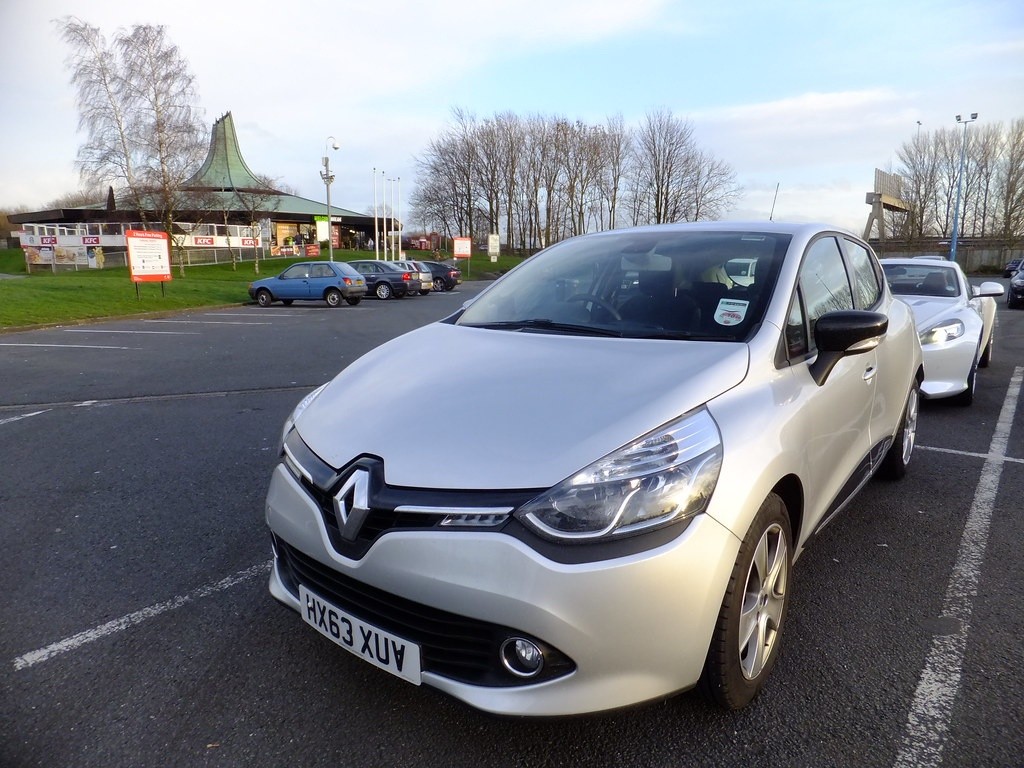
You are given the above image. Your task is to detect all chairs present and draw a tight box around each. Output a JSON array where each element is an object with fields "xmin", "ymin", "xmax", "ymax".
[
  {"xmin": 611, "ymin": 250, "xmax": 786, "ymax": 336},
  {"xmin": 919, "ymin": 271, "xmax": 948, "ymax": 295}
]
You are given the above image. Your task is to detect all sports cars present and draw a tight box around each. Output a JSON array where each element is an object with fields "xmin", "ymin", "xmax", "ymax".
[
  {"xmin": 708, "ymin": 255, "xmax": 1006, "ymax": 403},
  {"xmin": 262, "ymin": 220, "xmax": 926, "ymax": 721}
]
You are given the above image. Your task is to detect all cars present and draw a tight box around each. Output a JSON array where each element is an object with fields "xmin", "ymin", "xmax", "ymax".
[
  {"xmin": 247, "ymin": 260, "xmax": 368, "ymax": 308},
  {"xmin": 1007, "ymin": 259, "xmax": 1024, "ymax": 309},
  {"xmin": 367, "ymin": 260, "xmax": 434, "ymax": 296},
  {"xmin": 421, "ymin": 261, "xmax": 462, "ymax": 292},
  {"xmin": 326, "ymin": 259, "xmax": 422, "ymax": 300},
  {"xmin": 1003, "ymin": 258, "xmax": 1024, "ymax": 278}
]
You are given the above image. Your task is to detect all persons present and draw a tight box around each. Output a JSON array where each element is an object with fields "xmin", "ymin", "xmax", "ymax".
[{"xmin": 368, "ymin": 238, "xmax": 373, "ymax": 251}]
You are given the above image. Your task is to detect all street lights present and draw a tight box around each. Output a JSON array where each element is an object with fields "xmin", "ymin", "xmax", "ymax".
[
  {"xmin": 946, "ymin": 113, "xmax": 978, "ymax": 262},
  {"xmin": 319, "ymin": 135, "xmax": 340, "ymax": 262}
]
